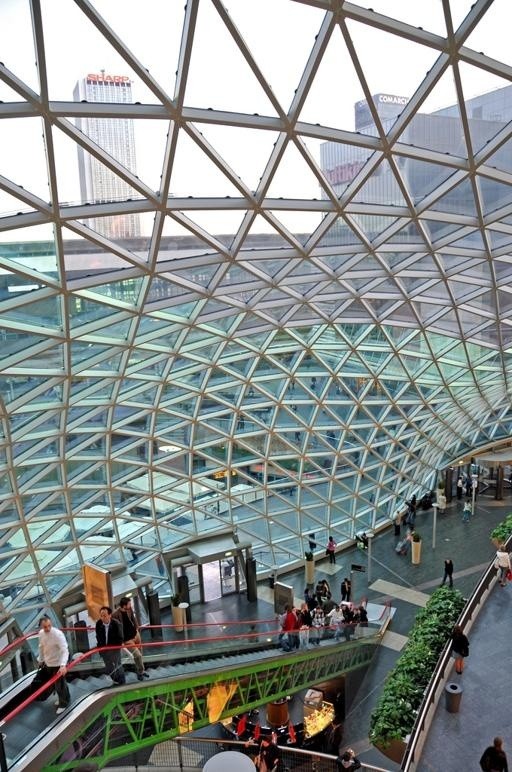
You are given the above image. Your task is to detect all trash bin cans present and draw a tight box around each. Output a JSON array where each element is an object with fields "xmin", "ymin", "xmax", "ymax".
[
  {"xmin": 444, "ymin": 682, "xmax": 463, "ymax": 713},
  {"xmin": 268, "ymin": 573, "xmax": 278, "ymax": 589}
]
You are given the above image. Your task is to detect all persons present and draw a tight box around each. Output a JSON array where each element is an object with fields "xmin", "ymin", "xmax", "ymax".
[
  {"xmin": 94, "ymin": 606, "xmax": 126, "ymax": 686},
  {"xmin": 245, "ymin": 730, "xmax": 284, "ymax": 772},
  {"xmin": 35, "ymin": 612, "xmax": 70, "ymax": 715},
  {"xmin": 479, "ymin": 735, "xmax": 509, "ymax": 772},
  {"xmin": 111, "ymin": 597, "xmax": 149, "ymax": 681},
  {"xmin": 440, "ymin": 560, "xmax": 453, "ymax": 588},
  {"xmin": 247, "ymin": 625, "xmax": 259, "ymax": 643},
  {"xmin": 308, "ymin": 533, "xmax": 316, "ymax": 552},
  {"xmin": 235, "ymin": 377, "xmax": 338, "ymax": 442},
  {"xmin": 496, "ymin": 545, "xmax": 511, "ymax": 587},
  {"xmin": 277, "ymin": 577, "xmax": 369, "ymax": 652},
  {"xmin": 356, "ymin": 531, "xmax": 368, "ymax": 549},
  {"xmin": 392, "ymin": 472, "xmax": 478, "ymax": 555},
  {"xmin": 451, "ymin": 624, "xmax": 469, "ymax": 675},
  {"xmin": 327, "ymin": 536, "xmax": 337, "ymax": 564},
  {"xmin": 335, "ymin": 748, "xmax": 361, "ymax": 772}
]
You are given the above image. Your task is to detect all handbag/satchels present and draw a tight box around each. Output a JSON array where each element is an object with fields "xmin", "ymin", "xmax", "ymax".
[{"xmin": 30, "ymin": 664, "xmax": 55, "ymax": 701}]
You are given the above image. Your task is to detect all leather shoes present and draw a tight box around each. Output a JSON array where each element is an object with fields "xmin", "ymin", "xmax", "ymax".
[
  {"xmin": 138, "ymin": 674, "xmax": 143, "ymax": 680},
  {"xmin": 143, "ymin": 672, "xmax": 150, "ymax": 677}
]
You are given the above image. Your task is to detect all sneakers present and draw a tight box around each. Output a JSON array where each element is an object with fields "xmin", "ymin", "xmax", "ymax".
[
  {"xmin": 55, "ymin": 700, "xmax": 60, "ymax": 705},
  {"xmin": 55, "ymin": 706, "xmax": 66, "ymax": 715}
]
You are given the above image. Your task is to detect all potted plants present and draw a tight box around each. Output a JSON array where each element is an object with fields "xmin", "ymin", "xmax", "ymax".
[
  {"xmin": 367, "ymin": 579, "xmax": 469, "ymax": 762},
  {"xmin": 304, "ymin": 547, "xmax": 316, "ymax": 587},
  {"xmin": 489, "ymin": 509, "xmax": 512, "ymax": 559},
  {"xmin": 412, "ymin": 530, "xmax": 422, "ymax": 564},
  {"xmin": 168, "ymin": 592, "xmax": 188, "ymax": 633}
]
